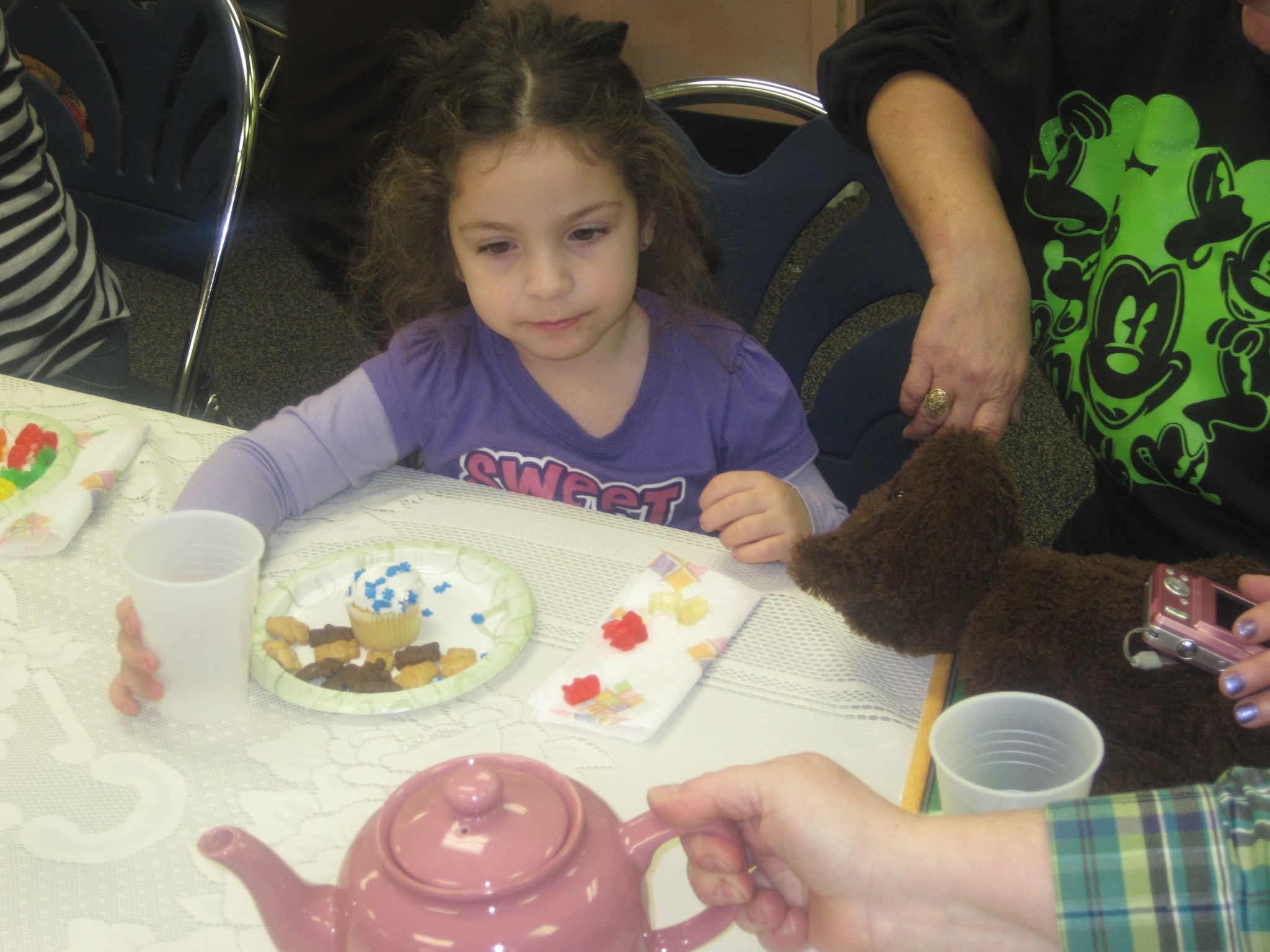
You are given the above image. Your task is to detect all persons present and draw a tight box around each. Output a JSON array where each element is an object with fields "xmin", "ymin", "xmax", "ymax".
[
  {"xmin": 646, "ymin": 752, "xmax": 1270, "ymax": 952},
  {"xmin": 277, "ymin": 2, "xmax": 484, "ymax": 295},
  {"xmin": 102, "ymin": 5, "xmax": 853, "ymax": 717},
  {"xmin": 1217, "ymin": 572, "xmax": 1270, "ymax": 730},
  {"xmin": 0, "ymin": 7, "xmax": 133, "ymax": 403},
  {"xmin": 815, "ymin": 0, "xmax": 1270, "ymax": 565}
]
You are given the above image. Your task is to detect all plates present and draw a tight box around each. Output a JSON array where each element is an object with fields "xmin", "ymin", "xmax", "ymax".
[{"xmin": 250, "ymin": 538, "xmax": 531, "ymax": 715}]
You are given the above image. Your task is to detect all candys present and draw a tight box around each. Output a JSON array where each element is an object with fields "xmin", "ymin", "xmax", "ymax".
[{"xmin": 0, "ymin": 422, "xmax": 59, "ymax": 502}]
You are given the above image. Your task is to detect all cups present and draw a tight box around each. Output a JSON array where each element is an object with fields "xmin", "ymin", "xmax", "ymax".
[
  {"xmin": 928, "ymin": 689, "xmax": 1103, "ymax": 812},
  {"xmin": 118, "ymin": 511, "xmax": 265, "ymax": 725}
]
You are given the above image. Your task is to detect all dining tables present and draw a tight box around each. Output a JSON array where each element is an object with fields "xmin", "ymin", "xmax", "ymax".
[{"xmin": 2, "ymin": 362, "xmax": 973, "ymax": 952}]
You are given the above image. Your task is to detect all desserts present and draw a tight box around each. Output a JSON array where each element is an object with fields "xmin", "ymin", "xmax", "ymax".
[{"xmin": 345, "ymin": 561, "xmax": 424, "ymax": 653}]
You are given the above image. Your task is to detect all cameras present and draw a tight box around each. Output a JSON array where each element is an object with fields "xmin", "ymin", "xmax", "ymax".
[{"xmin": 1138, "ymin": 562, "xmax": 1270, "ymax": 677}]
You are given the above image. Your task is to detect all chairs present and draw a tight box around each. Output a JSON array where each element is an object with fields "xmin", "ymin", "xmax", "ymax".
[
  {"xmin": 10, "ymin": 2, "xmax": 266, "ymax": 423},
  {"xmin": 635, "ymin": 73, "xmax": 934, "ymax": 521}
]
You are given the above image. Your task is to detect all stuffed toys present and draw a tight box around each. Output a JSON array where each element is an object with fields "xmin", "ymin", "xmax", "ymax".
[{"xmin": 787, "ymin": 428, "xmax": 1270, "ymax": 799}]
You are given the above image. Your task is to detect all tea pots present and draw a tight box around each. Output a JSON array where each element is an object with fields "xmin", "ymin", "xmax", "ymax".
[{"xmin": 200, "ymin": 751, "xmax": 751, "ymax": 952}]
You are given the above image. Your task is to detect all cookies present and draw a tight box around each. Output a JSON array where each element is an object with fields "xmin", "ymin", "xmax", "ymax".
[{"xmin": 261, "ymin": 616, "xmax": 477, "ymax": 694}]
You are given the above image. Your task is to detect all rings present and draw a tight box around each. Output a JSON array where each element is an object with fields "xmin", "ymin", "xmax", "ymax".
[{"xmin": 924, "ymin": 388, "xmax": 952, "ymax": 418}]
